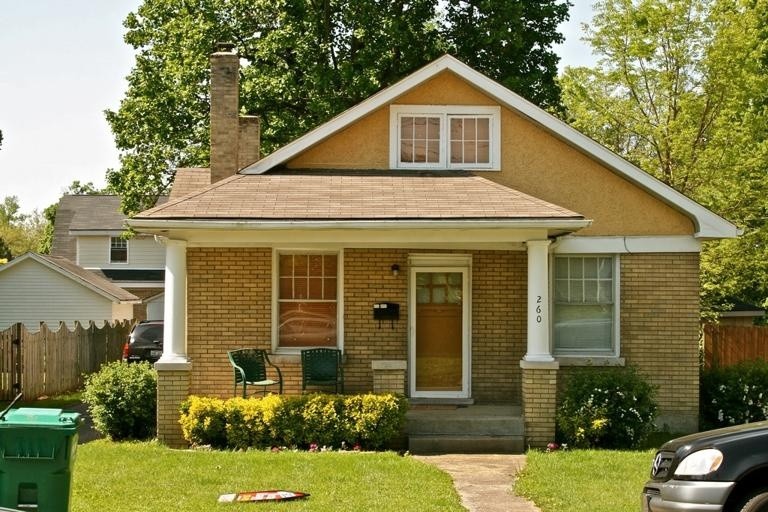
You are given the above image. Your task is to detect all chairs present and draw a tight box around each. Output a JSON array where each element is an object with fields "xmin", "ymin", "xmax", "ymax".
[
  {"xmin": 227, "ymin": 348, "xmax": 282, "ymax": 399},
  {"xmin": 301, "ymin": 347, "xmax": 345, "ymax": 395}
]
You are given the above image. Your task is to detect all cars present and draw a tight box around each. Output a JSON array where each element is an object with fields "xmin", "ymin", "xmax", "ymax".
[{"xmin": 642, "ymin": 420, "xmax": 768, "ymax": 512}]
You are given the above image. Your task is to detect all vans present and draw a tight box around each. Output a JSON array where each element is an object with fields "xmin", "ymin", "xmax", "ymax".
[{"xmin": 122, "ymin": 320, "xmax": 163, "ymax": 364}]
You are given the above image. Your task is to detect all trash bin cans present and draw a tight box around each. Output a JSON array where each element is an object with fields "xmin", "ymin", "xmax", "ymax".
[{"xmin": 0, "ymin": 408, "xmax": 81, "ymax": 512}]
